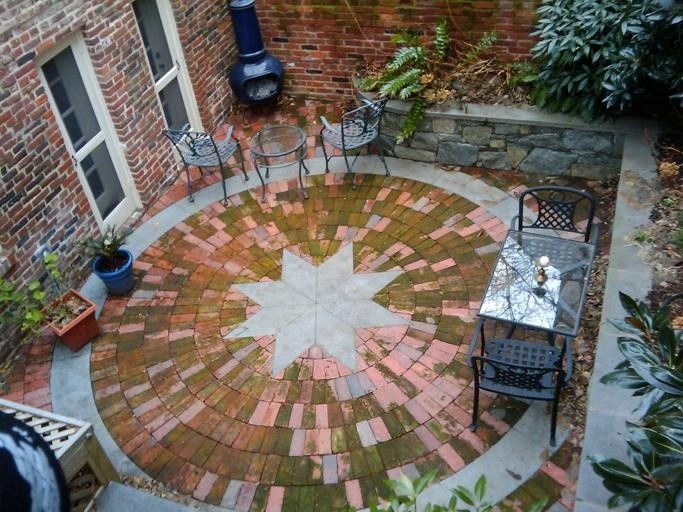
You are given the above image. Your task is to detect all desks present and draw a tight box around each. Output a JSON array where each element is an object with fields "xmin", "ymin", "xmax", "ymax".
[
  {"xmin": 245, "ymin": 123, "xmax": 314, "ymax": 204},
  {"xmin": 477, "ymin": 228, "xmax": 597, "ymax": 394}
]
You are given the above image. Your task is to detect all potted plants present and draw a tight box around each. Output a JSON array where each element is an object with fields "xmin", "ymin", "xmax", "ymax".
[{"xmin": 0, "ymin": 223, "xmax": 138, "ymax": 353}]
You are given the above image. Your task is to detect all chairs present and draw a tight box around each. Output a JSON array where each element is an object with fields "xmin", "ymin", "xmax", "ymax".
[
  {"xmin": 462, "ymin": 316, "xmax": 576, "ymax": 452},
  {"xmin": 161, "ymin": 122, "xmax": 250, "ymax": 206},
  {"xmin": 318, "ymin": 96, "xmax": 393, "ymax": 189},
  {"xmin": 508, "ymin": 184, "xmax": 604, "ymax": 271}
]
